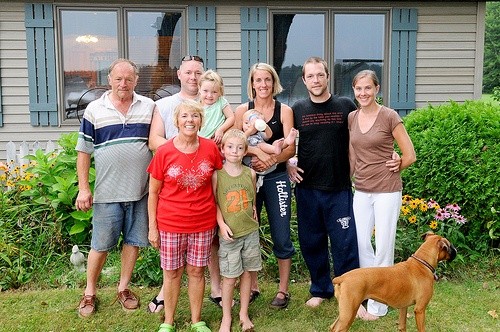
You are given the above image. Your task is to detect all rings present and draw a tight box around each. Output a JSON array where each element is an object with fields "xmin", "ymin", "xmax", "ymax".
[{"xmin": 261, "ymin": 170, "xmax": 263, "ymax": 172}]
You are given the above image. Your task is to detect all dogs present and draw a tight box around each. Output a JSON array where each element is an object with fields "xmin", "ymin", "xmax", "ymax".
[{"xmin": 328, "ymin": 230, "xmax": 458, "ymax": 332}]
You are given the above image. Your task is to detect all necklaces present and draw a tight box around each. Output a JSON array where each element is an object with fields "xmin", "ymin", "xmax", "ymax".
[{"xmin": 181, "ymin": 143, "xmax": 198, "ymax": 164}]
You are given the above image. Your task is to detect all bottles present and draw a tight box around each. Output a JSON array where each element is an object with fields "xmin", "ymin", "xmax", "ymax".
[{"xmin": 248, "ymin": 112, "xmax": 266, "ymax": 131}]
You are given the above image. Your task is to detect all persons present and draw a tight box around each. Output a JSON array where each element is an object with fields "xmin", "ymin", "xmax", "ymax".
[
  {"xmin": 226, "ymin": 63, "xmax": 296, "ymax": 310},
  {"xmin": 74, "ymin": 58, "xmax": 158, "ymax": 319},
  {"xmin": 195, "ymin": 69, "xmax": 234, "ymax": 149},
  {"xmin": 148, "ymin": 54, "xmax": 234, "ymax": 313},
  {"xmin": 348, "ymin": 69, "xmax": 417, "ymax": 316},
  {"xmin": 291, "ymin": 56, "xmax": 402, "ymax": 319},
  {"xmin": 212, "ymin": 129, "xmax": 260, "ymax": 332},
  {"xmin": 242, "ymin": 108, "xmax": 298, "ymax": 175},
  {"xmin": 145, "ymin": 99, "xmax": 224, "ymax": 332}
]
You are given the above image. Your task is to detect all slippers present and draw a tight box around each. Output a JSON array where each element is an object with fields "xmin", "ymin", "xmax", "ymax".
[
  {"xmin": 157, "ymin": 322, "xmax": 177, "ymax": 332},
  {"xmin": 148, "ymin": 295, "xmax": 165, "ymax": 314},
  {"xmin": 210, "ymin": 294, "xmax": 235, "ymax": 309},
  {"xmin": 188, "ymin": 321, "xmax": 212, "ymax": 332}
]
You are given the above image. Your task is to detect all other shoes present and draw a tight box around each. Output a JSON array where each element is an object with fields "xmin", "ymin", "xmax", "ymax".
[
  {"xmin": 269, "ymin": 290, "xmax": 290, "ymax": 309},
  {"xmin": 110, "ymin": 288, "xmax": 141, "ymax": 313},
  {"xmin": 74, "ymin": 292, "xmax": 99, "ymax": 320},
  {"xmin": 239, "ymin": 289, "xmax": 260, "ymax": 305}
]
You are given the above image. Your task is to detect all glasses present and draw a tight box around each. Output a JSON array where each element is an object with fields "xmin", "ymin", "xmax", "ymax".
[{"xmin": 181, "ymin": 55, "xmax": 204, "ymax": 66}]
